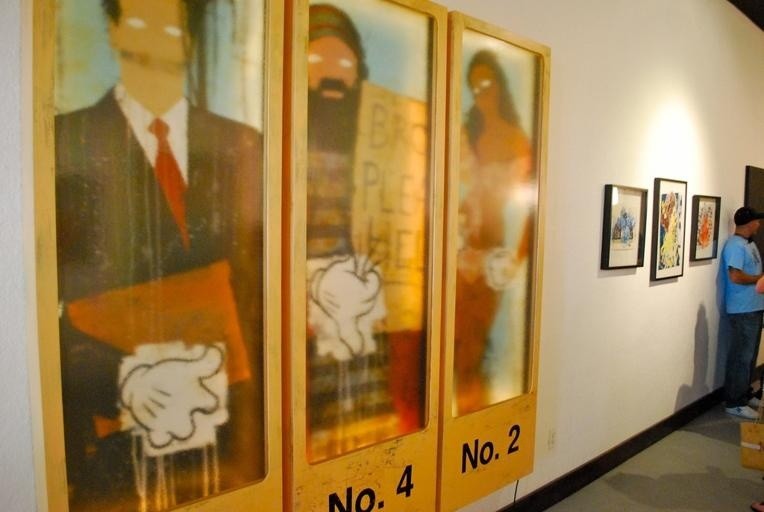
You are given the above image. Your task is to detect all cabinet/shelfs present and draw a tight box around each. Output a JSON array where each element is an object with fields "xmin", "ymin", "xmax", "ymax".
[{"xmin": 596, "ymin": 177, "xmax": 721, "ymax": 282}]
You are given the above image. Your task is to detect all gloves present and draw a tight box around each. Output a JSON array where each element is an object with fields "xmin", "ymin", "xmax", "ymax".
[
  {"xmin": 309, "ymin": 256, "xmax": 381, "ymax": 357},
  {"xmin": 490, "ymin": 249, "xmax": 518, "ymax": 286},
  {"xmin": 121, "ymin": 344, "xmax": 222, "ymax": 448}
]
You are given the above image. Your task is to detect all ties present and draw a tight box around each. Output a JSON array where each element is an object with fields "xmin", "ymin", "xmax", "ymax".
[{"xmin": 146, "ymin": 116, "xmax": 190, "ymax": 256}]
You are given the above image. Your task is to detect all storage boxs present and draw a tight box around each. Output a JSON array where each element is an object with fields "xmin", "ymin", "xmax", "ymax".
[{"xmin": 739, "ymin": 419, "xmax": 764, "ymax": 470}]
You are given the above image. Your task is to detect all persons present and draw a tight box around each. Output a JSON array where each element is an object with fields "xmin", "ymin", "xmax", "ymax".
[{"xmin": 721, "ymin": 205, "xmax": 764, "ymax": 422}]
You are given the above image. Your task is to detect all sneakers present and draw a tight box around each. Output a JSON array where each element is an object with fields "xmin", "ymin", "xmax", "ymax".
[
  {"xmin": 747, "ymin": 397, "xmax": 764, "ymax": 407},
  {"xmin": 725, "ymin": 405, "xmax": 761, "ymax": 419}
]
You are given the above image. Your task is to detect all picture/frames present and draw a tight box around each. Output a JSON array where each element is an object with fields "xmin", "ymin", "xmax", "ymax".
[
  {"xmin": 438, "ymin": 10, "xmax": 551, "ymax": 511},
  {"xmin": 27, "ymin": 1, "xmax": 295, "ymax": 511},
  {"xmin": 286, "ymin": 0, "xmax": 450, "ymax": 511}
]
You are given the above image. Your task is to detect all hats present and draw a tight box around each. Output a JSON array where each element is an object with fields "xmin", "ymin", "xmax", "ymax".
[
  {"xmin": 309, "ymin": 3, "xmax": 363, "ymax": 65},
  {"xmin": 734, "ymin": 206, "xmax": 764, "ymax": 225}
]
